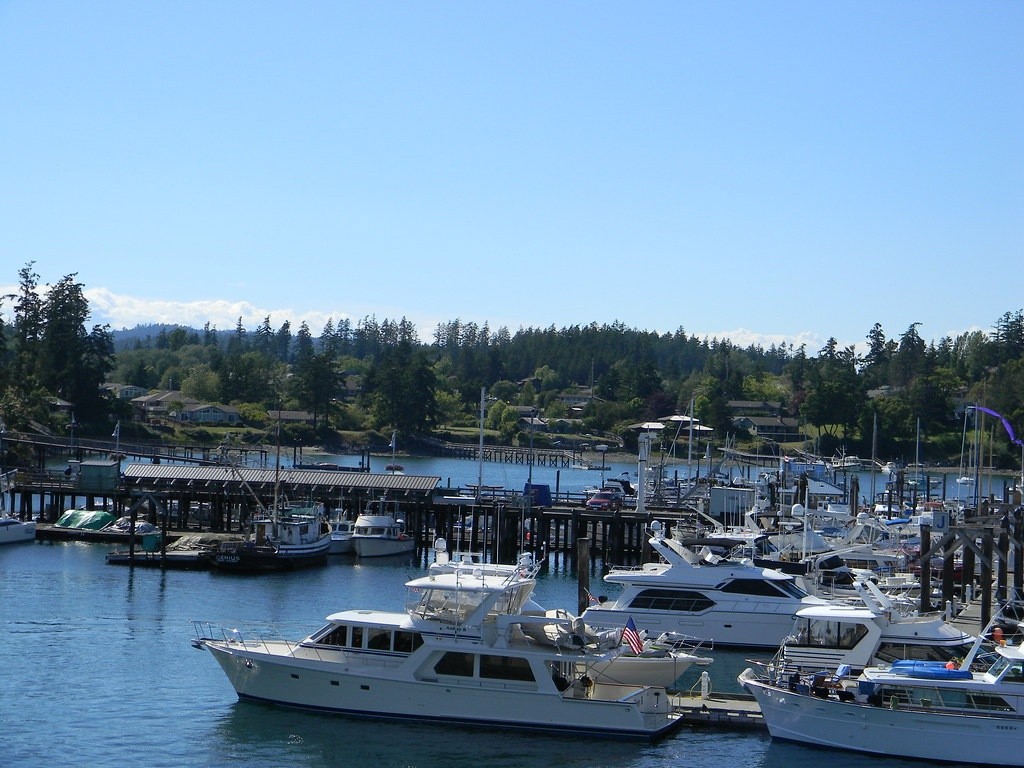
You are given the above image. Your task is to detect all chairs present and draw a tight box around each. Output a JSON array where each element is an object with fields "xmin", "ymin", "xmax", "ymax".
[
  {"xmin": 770, "ymin": 660, "xmax": 875, "ymax": 705},
  {"xmin": 553, "ymin": 618, "xmax": 676, "ymax": 658}
]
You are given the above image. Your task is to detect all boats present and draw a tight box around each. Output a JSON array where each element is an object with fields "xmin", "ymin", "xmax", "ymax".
[{"xmin": 0, "ymin": 380, "xmax": 1022, "ymax": 767}]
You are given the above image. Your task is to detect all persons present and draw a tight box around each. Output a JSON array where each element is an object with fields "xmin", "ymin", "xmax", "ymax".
[
  {"xmin": 64, "ymin": 465, "xmax": 73, "ymax": 482},
  {"xmin": 287, "ymin": 526, "xmax": 292, "ymax": 545},
  {"xmin": 797, "ymin": 626, "xmax": 807, "ymax": 645},
  {"xmin": 821, "ymin": 629, "xmax": 835, "ymax": 646},
  {"xmin": 807, "ymin": 630, "xmax": 814, "ymax": 644}
]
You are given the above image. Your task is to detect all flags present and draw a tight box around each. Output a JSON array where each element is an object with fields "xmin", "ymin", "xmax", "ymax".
[
  {"xmin": 622, "ymin": 615, "xmax": 644, "ymax": 655},
  {"xmin": 389, "ymin": 434, "xmax": 394, "ymax": 447},
  {"xmin": 586, "ymin": 589, "xmax": 599, "ymax": 605},
  {"xmin": 112, "ymin": 422, "xmax": 118, "ymax": 437}
]
